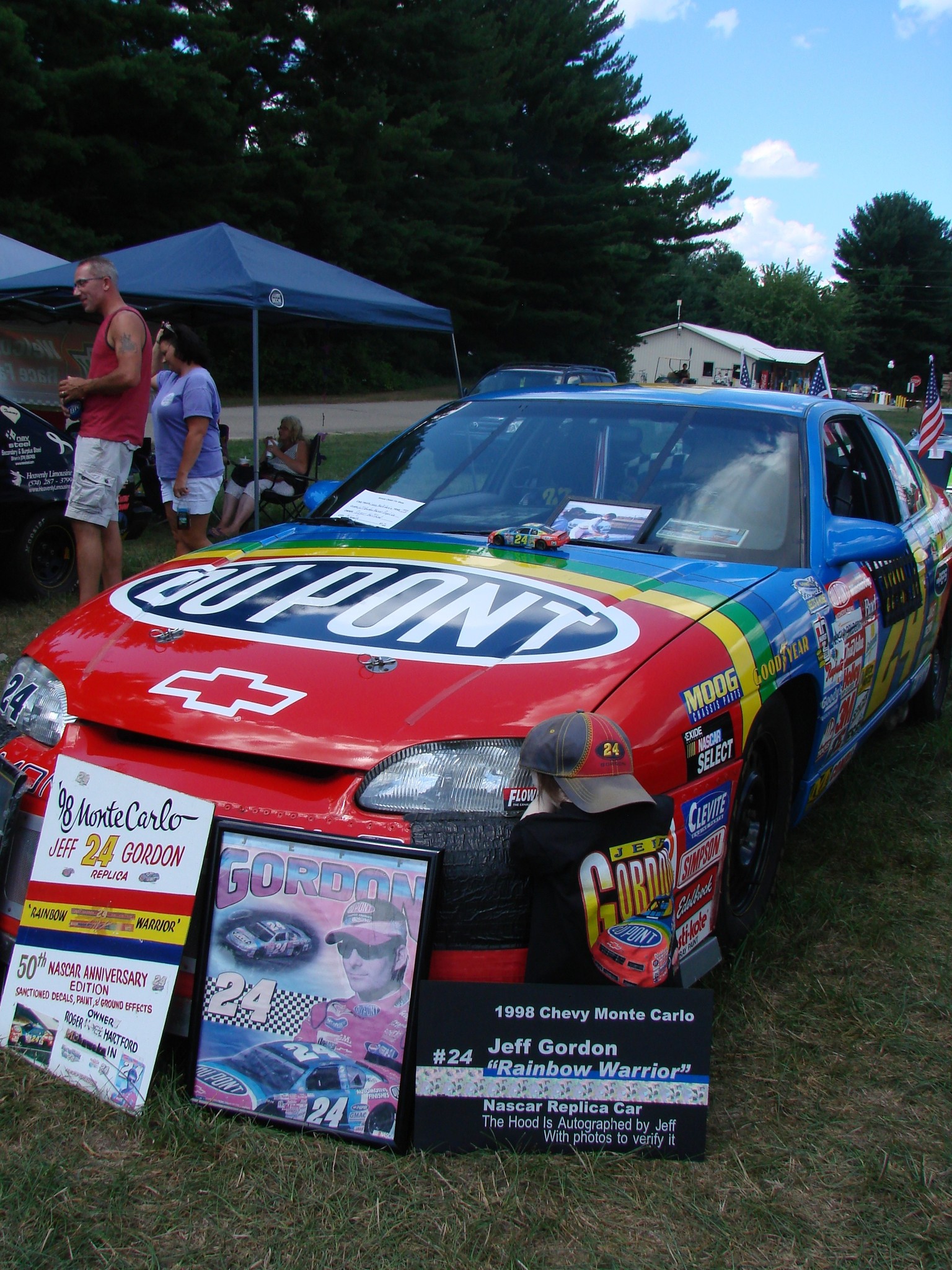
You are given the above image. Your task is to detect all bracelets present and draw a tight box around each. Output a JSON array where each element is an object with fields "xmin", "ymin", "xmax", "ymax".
[{"xmin": 156, "ymin": 341, "xmax": 160, "ymax": 343}]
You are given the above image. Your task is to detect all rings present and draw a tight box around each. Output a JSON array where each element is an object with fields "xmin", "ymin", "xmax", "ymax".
[
  {"xmin": 62, "ymin": 391, "xmax": 64, "ymax": 396},
  {"xmin": 181, "ymin": 492, "xmax": 184, "ymax": 495}
]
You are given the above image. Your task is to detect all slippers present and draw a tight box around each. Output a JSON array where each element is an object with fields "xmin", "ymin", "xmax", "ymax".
[{"xmin": 209, "ymin": 527, "xmax": 231, "ymax": 540}]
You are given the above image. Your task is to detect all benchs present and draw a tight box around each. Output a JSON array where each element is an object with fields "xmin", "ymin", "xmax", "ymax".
[{"xmin": 667, "ymin": 373, "xmax": 690, "ymax": 379}]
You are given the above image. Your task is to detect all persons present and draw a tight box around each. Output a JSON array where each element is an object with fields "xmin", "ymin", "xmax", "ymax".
[
  {"xmin": 569, "ymin": 513, "xmax": 617, "ymax": 540},
  {"xmin": 209, "ymin": 415, "xmax": 309, "ymax": 538},
  {"xmin": 149, "ymin": 321, "xmax": 225, "ymax": 554},
  {"xmin": 111, "ymin": 1068, "xmax": 137, "ymax": 1111},
  {"xmin": 551, "ymin": 507, "xmax": 586, "ymax": 531},
  {"xmin": 724, "ymin": 373, "xmax": 728, "ymax": 383},
  {"xmin": 58, "ymin": 255, "xmax": 153, "ymax": 608},
  {"xmin": 714, "ymin": 371, "xmax": 721, "ymax": 381},
  {"xmin": 797, "ymin": 374, "xmax": 803, "ymax": 392},
  {"xmin": 297, "ymin": 898, "xmax": 410, "ymax": 1071},
  {"xmin": 676, "ymin": 364, "xmax": 690, "ymax": 384}
]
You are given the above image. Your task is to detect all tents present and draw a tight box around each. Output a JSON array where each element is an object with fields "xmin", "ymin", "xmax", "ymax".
[{"xmin": 0, "ymin": 221, "xmax": 463, "ymax": 532}]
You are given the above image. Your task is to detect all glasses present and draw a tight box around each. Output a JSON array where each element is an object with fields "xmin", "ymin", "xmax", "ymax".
[
  {"xmin": 278, "ymin": 426, "xmax": 293, "ymax": 431},
  {"xmin": 75, "ymin": 275, "xmax": 111, "ymax": 288},
  {"xmin": 161, "ymin": 322, "xmax": 175, "ymax": 333}
]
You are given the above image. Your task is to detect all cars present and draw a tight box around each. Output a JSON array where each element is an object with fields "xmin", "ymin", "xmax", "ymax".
[
  {"xmin": 903, "ymin": 409, "xmax": 952, "ymax": 486},
  {"xmin": 0, "ymin": 389, "xmax": 952, "ymax": 1010},
  {"xmin": 847, "ymin": 384, "xmax": 874, "ymax": 402}
]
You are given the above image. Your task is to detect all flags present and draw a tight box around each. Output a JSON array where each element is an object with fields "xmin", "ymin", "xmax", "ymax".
[
  {"xmin": 808, "ymin": 359, "xmax": 840, "ymax": 447},
  {"xmin": 917, "ymin": 360, "xmax": 945, "ymax": 458},
  {"xmin": 739, "ymin": 353, "xmax": 750, "ymax": 388}
]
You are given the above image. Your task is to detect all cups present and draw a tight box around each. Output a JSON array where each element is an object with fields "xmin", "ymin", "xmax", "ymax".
[
  {"xmin": 239, "ymin": 458, "xmax": 251, "ymax": 467},
  {"xmin": 266, "ymin": 439, "xmax": 279, "ymax": 459}
]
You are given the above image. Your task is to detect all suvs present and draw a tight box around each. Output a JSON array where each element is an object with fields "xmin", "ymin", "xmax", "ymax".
[{"xmin": 431, "ymin": 364, "xmax": 618, "ymax": 472}]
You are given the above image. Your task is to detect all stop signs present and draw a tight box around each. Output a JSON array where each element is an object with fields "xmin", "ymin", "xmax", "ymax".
[{"xmin": 910, "ymin": 375, "xmax": 920, "ymax": 387}]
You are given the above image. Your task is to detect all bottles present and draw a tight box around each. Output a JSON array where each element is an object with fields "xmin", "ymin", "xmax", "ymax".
[
  {"xmin": 65, "ymin": 397, "xmax": 82, "ymax": 421},
  {"xmin": 177, "ymin": 494, "xmax": 191, "ymax": 530}
]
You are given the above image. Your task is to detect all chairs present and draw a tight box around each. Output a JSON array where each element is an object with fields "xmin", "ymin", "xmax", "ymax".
[{"xmin": 259, "ymin": 432, "xmax": 327, "ymax": 525}]
